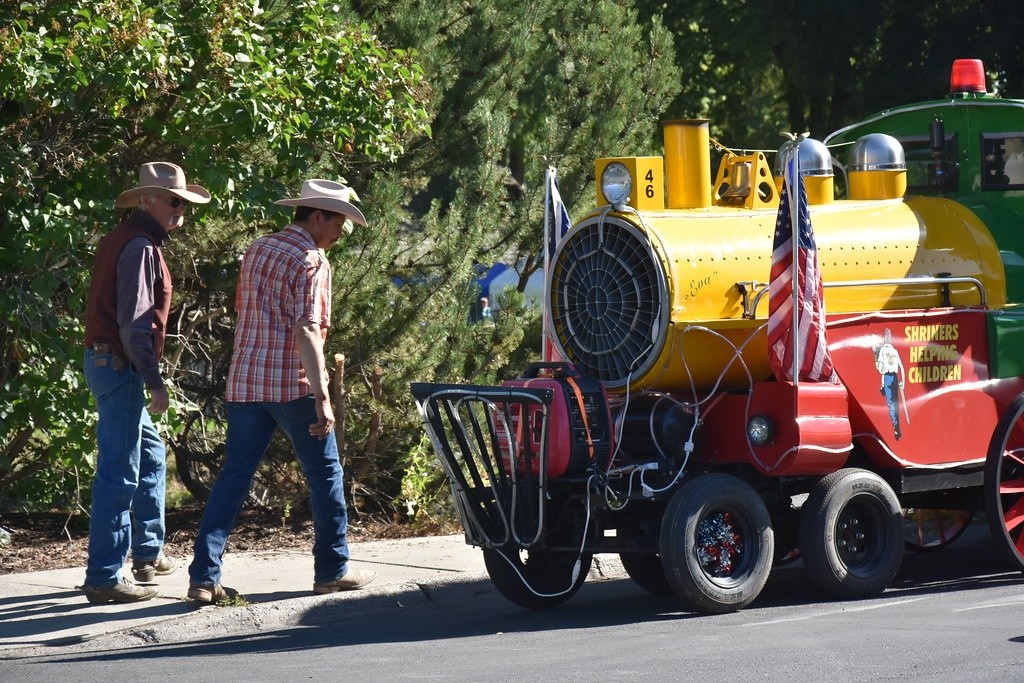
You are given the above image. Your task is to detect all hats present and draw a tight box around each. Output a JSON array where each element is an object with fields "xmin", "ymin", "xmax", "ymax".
[
  {"xmin": 274, "ymin": 179, "xmax": 369, "ymax": 229},
  {"xmin": 115, "ymin": 162, "xmax": 212, "ymax": 209}
]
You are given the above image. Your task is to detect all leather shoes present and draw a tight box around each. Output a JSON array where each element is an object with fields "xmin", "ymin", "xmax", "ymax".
[
  {"xmin": 313, "ymin": 568, "xmax": 377, "ymax": 594},
  {"xmin": 187, "ymin": 581, "xmax": 238, "ymax": 604}
]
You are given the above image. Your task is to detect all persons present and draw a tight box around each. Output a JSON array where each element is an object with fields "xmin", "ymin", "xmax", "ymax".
[
  {"xmin": 188, "ymin": 180, "xmax": 377, "ymax": 604},
  {"xmin": 482, "ymin": 298, "xmax": 491, "ymax": 319},
  {"xmin": 82, "ymin": 161, "xmax": 211, "ymax": 604}
]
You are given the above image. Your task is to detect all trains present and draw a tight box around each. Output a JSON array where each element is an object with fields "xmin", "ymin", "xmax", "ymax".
[{"xmin": 411, "ymin": 58, "xmax": 1024, "ymax": 616}]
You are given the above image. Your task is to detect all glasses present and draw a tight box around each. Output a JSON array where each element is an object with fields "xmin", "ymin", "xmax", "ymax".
[{"xmin": 156, "ymin": 195, "xmax": 188, "ymax": 208}]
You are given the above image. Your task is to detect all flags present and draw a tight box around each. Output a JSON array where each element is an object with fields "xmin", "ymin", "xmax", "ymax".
[
  {"xmin": 545, "ymin": 169, "xmax": 580, "ymax": 379},
  {"xmin": 766, "ymin": 143, "xmax": 842, "ymax": 385}
]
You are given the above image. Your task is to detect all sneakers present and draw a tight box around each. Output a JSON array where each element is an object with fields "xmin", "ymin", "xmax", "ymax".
[
  {"xmin": 84, "ymin": 577, "xmax": 159, "ymax": 603},
  {"xmin": 131, "ymin": 555, "xmax": 178, "ymax": 582}
]
